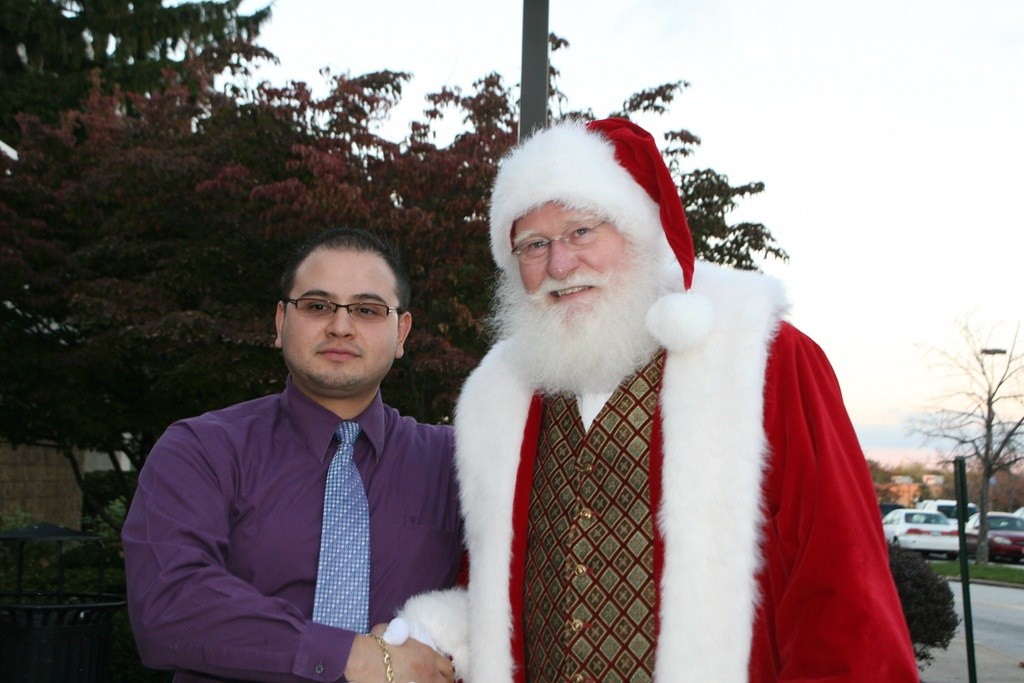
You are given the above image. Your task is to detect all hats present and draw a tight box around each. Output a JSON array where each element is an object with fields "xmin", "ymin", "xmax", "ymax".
[{"xmin": 488, "ymin": 114, "xmax": 713, "ymax": 352}]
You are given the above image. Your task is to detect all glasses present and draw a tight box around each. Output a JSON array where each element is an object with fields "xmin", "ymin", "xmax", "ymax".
[
  {"xmin": 284, "ymin": 297, "xmax": 398, "ymax": 324},
  {"xmin": 510, "ymin": 220, "xmax": 606, "ymax": 266}
]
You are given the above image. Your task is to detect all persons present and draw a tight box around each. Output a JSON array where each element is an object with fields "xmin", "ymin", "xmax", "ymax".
[
  {"xmin": 119, "ymin": 231, "xmax": 467, "ymax": 683},
  {"xmin": 382, "ymin": 116, "xmax": 919, "ymax": 683}
]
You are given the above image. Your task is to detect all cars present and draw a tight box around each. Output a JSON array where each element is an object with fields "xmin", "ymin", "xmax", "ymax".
[
  {"xmin": 963, "ymin": 511, "xmax": 1024, "ymax": 561},
  {"xmin": 879, "ymin": 508, "xmax": 959, "ymax": 560},
  {"xmin": 912, "ymin": 498, "xmax": 979, "ymax": 526}
]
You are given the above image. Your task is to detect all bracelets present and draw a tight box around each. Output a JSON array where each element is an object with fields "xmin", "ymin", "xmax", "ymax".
[{"xmin": 365, "ymin": 633, "xmax": 394, "ymax": 683}]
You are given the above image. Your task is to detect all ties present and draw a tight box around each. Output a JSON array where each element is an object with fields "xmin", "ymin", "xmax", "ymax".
[{"xmin": 312, "ymin": 421, "xmax": 371, "ymax": 635}]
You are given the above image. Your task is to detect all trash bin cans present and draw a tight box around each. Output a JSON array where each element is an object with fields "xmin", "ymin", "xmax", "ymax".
[{"xmin": 0, "ymin": 521, "xmax": 131, "ymax": 683}]
